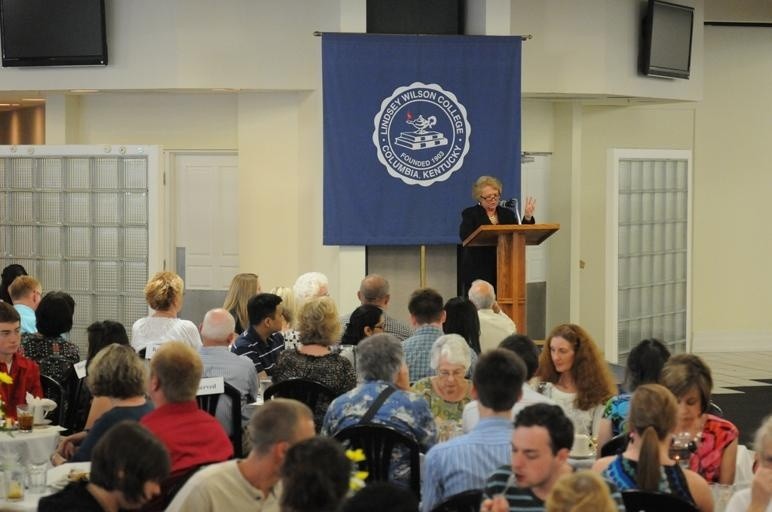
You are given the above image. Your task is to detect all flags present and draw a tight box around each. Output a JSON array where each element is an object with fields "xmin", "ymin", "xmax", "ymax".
[{"xmin": 320, "ymin": 33, "xmax": 522, "ymax": 245}]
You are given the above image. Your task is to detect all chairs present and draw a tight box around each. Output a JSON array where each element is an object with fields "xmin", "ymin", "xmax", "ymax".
[
  {"xmin": 41, "ymin": 373, "xmax": 64, "ymax": 429},
  {"xmin": 612, "ymin": 482, "xmax": 695, "ymax": 511},
  {"xmin": 328, "ymin": 420, "xmax": 422, "ymax": 500},
  {"xmin": 598, "ymin": 429, "xmax": 632, "ymax": 456},
  {"xmin": 262, "ymin": 375, "xmax": 341, "ymax": 438},
  {"xmin": 425, "ymin": 482, "xmax": 547, "ymax": 511},
  {"xmin": 193, "ymin": 379, "xmax": 243, "ymax": 459}
]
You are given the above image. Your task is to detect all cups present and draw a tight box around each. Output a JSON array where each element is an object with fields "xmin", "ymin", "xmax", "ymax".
[
  {"xmin": 5, "ymin": 464, "xmax": 25, "ymax": 502},
  {"xmin": 572, "ymin": 433, "xmax": 593, "ymax": 452},
  {"xmin": 17, "ymin": 403, "xmax": 35, "ymax": 434},
  {"xmin": 32, "ymin": 396, "xmax": 49, "ymax": 421},
  {"xmin": 25, "ymin": 457, "xmax": 49, "ymax": 494},
  {"xmin": 259, "ymin": 380, "xmax": 273, "ymax": 398},
  {"xmin": 707, "ymin": 478, "xmax": 734, "ymax": 511}
]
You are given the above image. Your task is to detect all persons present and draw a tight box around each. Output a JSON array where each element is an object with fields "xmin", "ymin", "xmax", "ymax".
[
  {"xmin": 162, "ymin": 398, "xmax": 352, "ymax": 511},
  {"xmin": 460, "ymin": 176, "xmax": 538, "ymax": 297},
  {"xmin": 324, "ymin": 324, "xmax": 772, "ymax": 512},
  {"xmin": 1, "ymin": 263, "xmax": 81, "ymax": 427},
  {"xmin": 31, "ymin": 420, "xmax": 170, "ymax": 512},
  {"xmin": 53, "ymin": 263, "xmax": 515, "ymax": 460}
]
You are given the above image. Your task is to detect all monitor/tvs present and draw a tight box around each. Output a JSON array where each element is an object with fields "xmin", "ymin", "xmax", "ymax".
[
  {"xmin": 365, "ymin": 0, "xmax": 464, "ymax": 34},
  {"xmin": 638, "ymin": 0, "xmax": 695, "ymax": 80},
  {"xmin": 0, "ymin": 0, "xmax": 108, "ymax": 67}
]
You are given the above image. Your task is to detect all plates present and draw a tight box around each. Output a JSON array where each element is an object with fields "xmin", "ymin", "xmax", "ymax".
[
  {"xmin": 565, "ymin": 452, "xmax": 594, "ymax": 460},
  {"xmin": 52, "ymin": 472, "xmax": 90, "ymax": 489},
  {"xmin": 33, "ymin": 419, "xmax": 54, "ymax": 425}
]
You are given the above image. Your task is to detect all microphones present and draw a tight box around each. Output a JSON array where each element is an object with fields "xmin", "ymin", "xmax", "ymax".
[{"xmin": 498, "ymin": 199, "xmax": 514, "ymax": 208}]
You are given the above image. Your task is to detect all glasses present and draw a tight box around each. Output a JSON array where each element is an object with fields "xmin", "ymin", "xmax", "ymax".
[
  {"xmin": 436, "ymin": 370, "xmax": 465, "ymax": 375},
  {"xmin": 373, "ymin": 323, "xmax": 387, "ymax": 330},
  {"xmin": 481, "ymin": 196, "xmax": 500, "ymax": 200}
]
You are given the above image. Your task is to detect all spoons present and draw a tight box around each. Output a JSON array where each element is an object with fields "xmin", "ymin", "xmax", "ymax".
[{"xmin": 500, "ymin": 474, "xmax": 519, "ymax": 498}]
